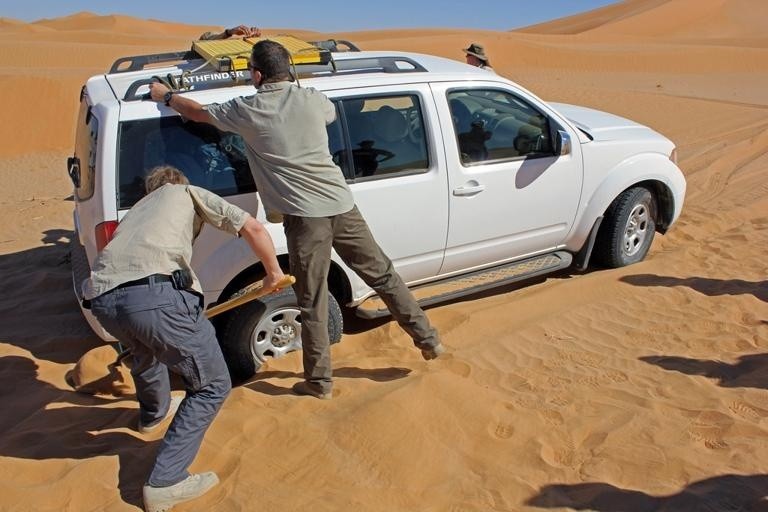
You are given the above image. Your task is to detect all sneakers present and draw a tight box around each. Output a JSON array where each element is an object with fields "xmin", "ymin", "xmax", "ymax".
[
  {"xmin": 138, "ymin": 396, "xmax": 183, "ymax": 432},
  {"xmin": 294, "ymin": 382, "xmax": 332, "ymax": 399},
  {"xmin": 142, "ymin": 472, "xmax": 219, "ymax": 512},
  {"xmin": 421, "ymin": 344, "xmax": 443, "ymax": 361}
]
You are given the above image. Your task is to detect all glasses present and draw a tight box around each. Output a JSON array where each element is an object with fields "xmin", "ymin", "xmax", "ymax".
[{"xmin": 247, "ymin": 62, "xmax": 259, "ymax": 70}]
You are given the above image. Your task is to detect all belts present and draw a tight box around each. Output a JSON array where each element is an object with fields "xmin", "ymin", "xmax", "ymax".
[{"xmin": 119, "ymin": 273, "xmax": 172, "ymax": 287}]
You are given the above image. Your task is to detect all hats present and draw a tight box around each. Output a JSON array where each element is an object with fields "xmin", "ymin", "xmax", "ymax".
[{"xmin": 462, "ymin": 43, "xmax": 488, "ymax": 62}]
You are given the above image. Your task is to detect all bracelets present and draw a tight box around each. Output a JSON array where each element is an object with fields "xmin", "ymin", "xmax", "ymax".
[{"xmin": 225, "ymin": 28, "xmax": 232, "ymax": 37}]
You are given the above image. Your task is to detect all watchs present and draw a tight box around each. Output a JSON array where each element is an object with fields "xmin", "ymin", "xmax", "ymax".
[{"xmin": 164, "ymin": 91, "xmax": 176, "ymax": 107}]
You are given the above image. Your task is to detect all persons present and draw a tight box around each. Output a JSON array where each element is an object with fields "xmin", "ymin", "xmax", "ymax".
[
  {"xmin": 147, "ymin": 39, "xmax": 446, "ymax": 399},
  {"xmin": 181, "ymin": 25, "xmax": 261, "ymax": 61},
  {"xmin": 80, "ymin": 166, "xmax": 286, "ymax": 512},
  {"xmin": 461, "ymin": 44, "xmax": 496, "ymax": 74}
]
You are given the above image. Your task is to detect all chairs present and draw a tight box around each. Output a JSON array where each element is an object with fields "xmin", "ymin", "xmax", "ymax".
[{"xmin": 371, "ymin": 104, "xmax": 415, "ymax": 171}]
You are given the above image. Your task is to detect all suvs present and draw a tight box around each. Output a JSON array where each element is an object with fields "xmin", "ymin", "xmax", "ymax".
[{"xmin": 66, "ymin": 38, "xmax": 689, "ymax": 379}]
